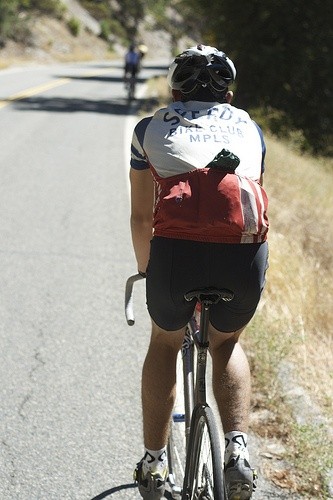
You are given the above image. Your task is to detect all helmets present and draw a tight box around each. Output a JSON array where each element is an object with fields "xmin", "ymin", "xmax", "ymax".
[{"xmin": 166, "ymin": 44, "xmax": 237, "ymax": 104}]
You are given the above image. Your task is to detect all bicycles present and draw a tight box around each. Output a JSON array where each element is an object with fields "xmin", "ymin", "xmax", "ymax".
[
  {"xmin": 124, "ymin": 272, "xmax": 253, "ymax": 500},
  {"xmin": 124, "ymin": 67, "xmax": 138, "ymax": 107}
]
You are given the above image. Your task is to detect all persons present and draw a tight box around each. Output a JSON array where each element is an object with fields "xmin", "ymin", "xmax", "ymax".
[
  {"xmin": 124, "ymin": 46, "xmax": 141, "ymax": 89},
  {"xmin": 129, "ymin": 44, "xmax": 270, "ymax": 500}
]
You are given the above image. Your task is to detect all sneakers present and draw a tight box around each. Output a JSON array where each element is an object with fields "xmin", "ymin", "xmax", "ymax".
[
  {"xmin": 223, "ymin": 450, "xmax": 257, "ymax": 500},
  {"xmin": 132, "ymin": 458, "xmax": 170, "ymax": 500}
]
